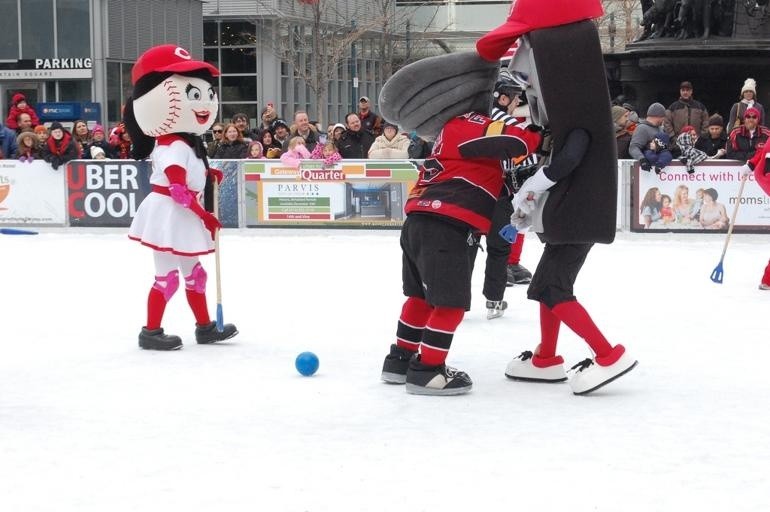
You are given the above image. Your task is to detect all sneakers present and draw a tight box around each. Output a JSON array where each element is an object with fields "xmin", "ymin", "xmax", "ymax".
[{"xmin": 486, "ymin": 300, "xmax": 507, "ymax": 310}]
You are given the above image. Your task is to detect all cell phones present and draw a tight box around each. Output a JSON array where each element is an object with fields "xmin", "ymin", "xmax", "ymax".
[{"xmin": 268, "ymin": 103, "xmax": 273, "ymax": 108}]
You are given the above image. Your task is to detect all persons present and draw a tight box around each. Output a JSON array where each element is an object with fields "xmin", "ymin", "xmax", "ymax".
[
  {"xmin": 484, "ymin": 67, "xmax": 548, "ymax": 319},
  {"xmin": 727, "ymin": 127, "xmax": 770, "ymax": 291},
  {"xmin": 506, "ymin": 226, "xmax": 531, "ymax": 285},
  {"xmin": 641, "ymin": 187, "xmax": 726, "ymax": 231}
]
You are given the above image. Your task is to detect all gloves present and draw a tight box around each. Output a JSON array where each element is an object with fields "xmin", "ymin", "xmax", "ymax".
[
  {"xmin": 640, "ymin": 158, "xmax": 652, "ymax": 172},
  {"xmin": 51, "ymin": 157, "xmax": 64, "ymax": 170}
]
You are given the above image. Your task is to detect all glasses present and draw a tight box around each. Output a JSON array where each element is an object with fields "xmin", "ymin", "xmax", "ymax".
[
  {"xmin": 746, "ymin": 115, "xmax": 757, "ymax": 120},
  {"xmin": 213, "ymin": 130, "xmax": 222, "ymax": 134}
]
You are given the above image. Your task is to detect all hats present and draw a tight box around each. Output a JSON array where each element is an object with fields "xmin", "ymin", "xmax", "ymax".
[
  {"xmin": 383, "ymin": 121, "xmax": 398, "ymax": 131},
  {"xmin": 613, "ymin": 106, "xmax": 627, "ymax": 122},
  {"xmin": 332, "ymin": 123, "xmax": 346, "ymax": 134},
  {"xmin": 359, "ymin": 95, "xmax": 368, "ymax": 102},
  {"xmin": 740, "ymin": 78, "xmax": 757, "ymax": 96},
  {"xmin": 13, "ymin": 93, "xmax": 25, "ymax": 106},
  {"xmin": 708, "ymin": 114, "xmax": 723, "ymax": 126},
  {"xmin": 89, "ymin": 146, "xmax": 105, "ymax": 158},
  {"xmin": 646, "ymin": 103, "xmax": 666, "ymax": 117},
  {"xmin": 680, "ymin": 82, "xmax": 693, "ymax": 90},
  {"xmin": 92, "ymin": 125, "xmax": 104, "ymax": 135}
]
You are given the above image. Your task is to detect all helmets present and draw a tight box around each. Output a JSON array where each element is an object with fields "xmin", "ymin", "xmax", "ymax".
[{"xmin": 495, "ymin": 67, "xmax": 528, "ymax": 99}]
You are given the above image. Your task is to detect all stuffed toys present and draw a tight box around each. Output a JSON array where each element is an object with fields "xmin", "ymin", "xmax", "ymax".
[
  {"xmin": 131, "ymin": 44, "xmax": 239, "ymax": 350},
  {"xmin": 377, "ymin": 51, "xmax": 544, "ymax": 394},
  {"xmin": 477, "ymin": 1, "xmax": 637, "ymax": 396}
]
